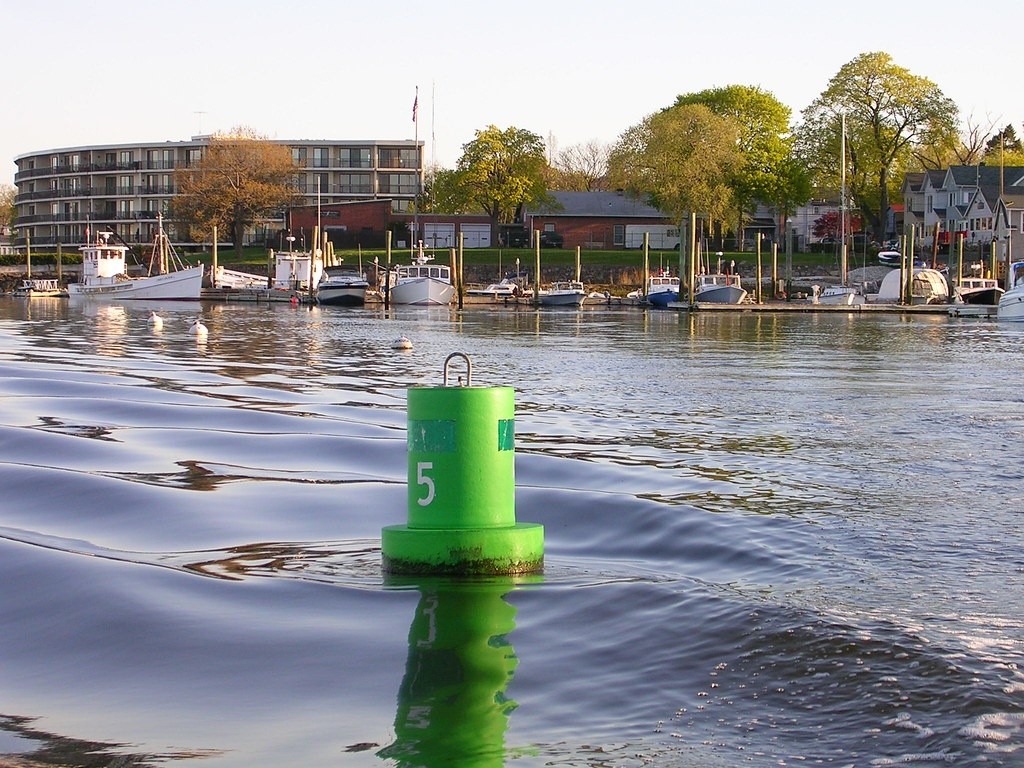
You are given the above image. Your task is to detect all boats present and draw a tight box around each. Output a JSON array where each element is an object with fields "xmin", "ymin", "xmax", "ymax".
[{"xmin": 14, "ymin": 215, "xmax": 1023, "ymax": 315}]
